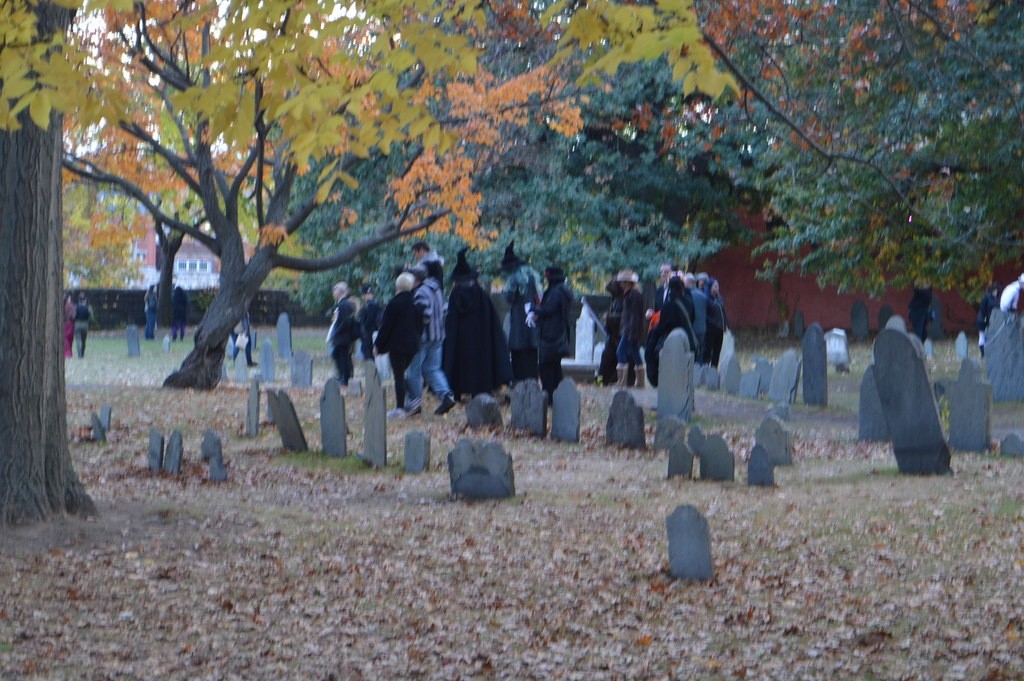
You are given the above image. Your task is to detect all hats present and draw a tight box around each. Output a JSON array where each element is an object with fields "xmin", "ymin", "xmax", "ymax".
[
  {"xmin": 617, "ymin": 270, "xmax": 641, "ymax": 284},
  {"xmin": 499, "ymin": 239, "xmax": 524, "ymax": 272},
  {"xmin": 546, "ymin": 266, "xmax": 564, "ymax": 281},
  {"xmin": 450, "ymin": 245, "xmax": 477, "ymax": 281}
]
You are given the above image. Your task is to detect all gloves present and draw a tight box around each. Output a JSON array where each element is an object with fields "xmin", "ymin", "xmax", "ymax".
[{"xmin": 524, "ymin": 302, "xmax": 538, "ymax": 327}]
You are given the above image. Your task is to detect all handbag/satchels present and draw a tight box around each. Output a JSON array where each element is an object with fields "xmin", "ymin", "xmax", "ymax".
[
  {"xmin": 236, "ymin": 334, "xmax": 249, "ymax": 351},
  {"xmin": 349, "ymin": 322, "xmax": 362, "ymax": 343}
]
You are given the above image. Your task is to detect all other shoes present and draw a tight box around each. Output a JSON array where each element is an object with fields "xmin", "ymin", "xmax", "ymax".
[
  {"xmin": 407, "ymin": 404, "xmax": 422, "ymax": 416},
  {"xmin": 435, "ymin": 395, "xmax": 455, "ymax": 416},
  {"xmin": 388, "ymin": 406, "xmax": 406, "ymax": 421},
  {"xmin": 247, "ymin": 361, "xmax": 258, "ymax": 368}
]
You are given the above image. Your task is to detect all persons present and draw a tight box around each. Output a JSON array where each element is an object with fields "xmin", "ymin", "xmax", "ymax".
[
  {"xmin": 975, "ymin": 272, "xmax": 1024, "ymax": 358},
  {"xmin": 170, "ymin": 286, "xmax": 188, "ymax": 342},
  {"xmin": 144, "ymin": 283, "xmax": 159, "ymax": 340},
  {"xmin": 231, "ymin": 312, "xmax": 257, "ymax": 367},
  {"xmin": 62, "ymin": 288, "xmax": 95, "ymax": 360},
  {"xmin": 597, "ymin": 264, "xmax": 729, "ymax": 388},
  {"xmin": 908, "ymin": 277, "xmax": 932, "ymax": 341},
  {"xmin": 325, "ymin": 241, "xmax": 573, "ymax": 421}
]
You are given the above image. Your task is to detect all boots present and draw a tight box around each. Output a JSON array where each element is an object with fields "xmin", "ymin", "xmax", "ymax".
[
  {"xmin": 607, "ymin": 364, "xmax": 628, "ymax": 391},
  {"xmin": 634, "ymin": 366, "xmax": 645, "ymax": 390}
]
